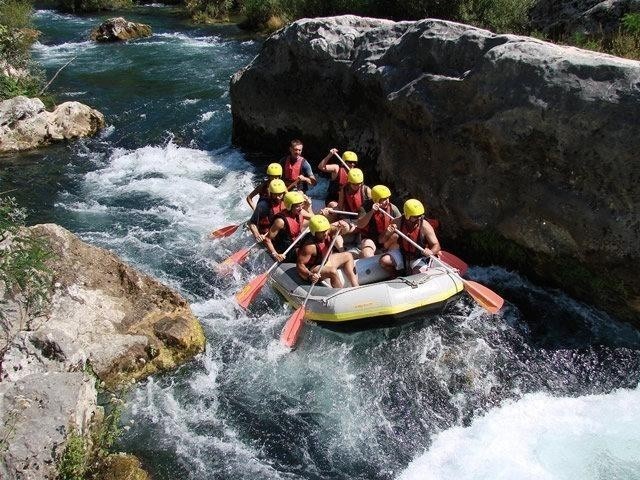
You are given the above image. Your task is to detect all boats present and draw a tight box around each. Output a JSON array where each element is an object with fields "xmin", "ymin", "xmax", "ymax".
[{"xmin": 243, "ymin": 200, "xmax": 467, "ymax": 333}]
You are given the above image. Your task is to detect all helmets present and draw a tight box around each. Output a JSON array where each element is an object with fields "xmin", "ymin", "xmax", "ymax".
[
  {"xmin": 309, "ymin": 215, "xmax": 331, "ymax": 237},
  {"xmin": 341, "ymin": 150, "xmax": 392, "ymax": 205},
  {"xmin": 403, "ymin": 198, "xmax": 425, "ymax": 221},
  {"xmin": 267, "ymin": 162, "xmax": 305, "ymax": 211}
]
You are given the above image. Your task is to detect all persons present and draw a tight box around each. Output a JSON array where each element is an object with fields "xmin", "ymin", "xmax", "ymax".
[{"xmin": 246, "ymin": 140, "xmax": 441, "ymax": 287}]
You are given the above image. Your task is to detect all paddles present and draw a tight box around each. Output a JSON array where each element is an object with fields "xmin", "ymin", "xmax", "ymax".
[
  {"xmin": 394, "ymin": 219, "xmax": 505, "ymax": 313},
  {"xmin": 208, "ymin": 219, "xmax": 341, "ymax": 346}
]
